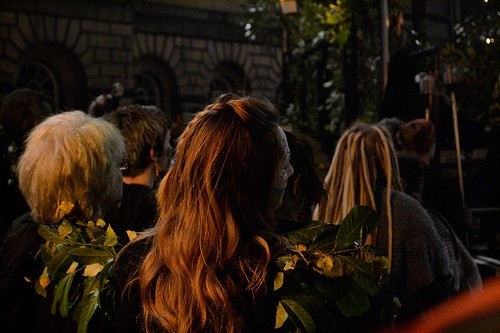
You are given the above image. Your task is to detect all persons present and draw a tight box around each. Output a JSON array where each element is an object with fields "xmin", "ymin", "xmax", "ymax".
[
  {"xmin": 271, "ymin": 116, "xmax": 483, "ymax": 321},
  {"xmin": 0, "ymin": 111, "xmax": 127, "ymax": 333},
  {"xmin": 62, "ymin": 93, "xmax": 389, "ymax": 333},
  {"xmin": 0, "ymin": 83, "xmax": 180, "ymax": 253}
]
